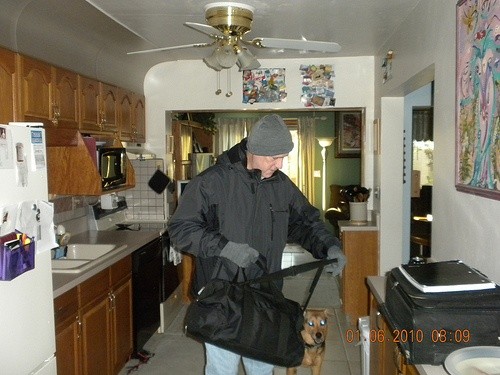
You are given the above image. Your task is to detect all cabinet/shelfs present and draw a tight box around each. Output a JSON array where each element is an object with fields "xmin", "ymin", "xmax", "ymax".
[
  {"xmin": 172, "ymin": 119, "xmax": 216, "ymax": 181},
  {"xmin": 369, "ymin": 290, "xmax": 420, "ymax": 375},
  {"xmin": 0, "ymin": 46, "xmax": 146, "ymax": 145},
  {"xmin": 338, "ymin": 222, "xmax": 379, "ymax": 317},
  {"xmin": 53, "ymin": 255, "xmax": 133, "ymax": 375}
]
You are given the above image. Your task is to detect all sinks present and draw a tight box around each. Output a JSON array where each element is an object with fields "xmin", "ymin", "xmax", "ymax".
[
  {"xmin": 51, "ymin": 260, "xmax": 91, "ymax": 273},
  {"xmin": 57, "ymin": 243, "xmax": 128, "ymax": 259}
]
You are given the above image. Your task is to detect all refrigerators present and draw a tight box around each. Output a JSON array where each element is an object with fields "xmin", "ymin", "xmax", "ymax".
[{"xmin": 0, "ymin": 123, "xmax": 60, "ymax": 375}]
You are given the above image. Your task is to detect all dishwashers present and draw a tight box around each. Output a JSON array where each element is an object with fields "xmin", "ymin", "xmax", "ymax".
[{"xmin": 131, "ymin": 240, "xmax": 160, "ymax": 353}]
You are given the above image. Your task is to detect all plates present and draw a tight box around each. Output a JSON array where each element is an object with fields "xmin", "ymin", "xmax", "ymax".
[{"xmin": 445, "ymin": 345, "xmax": 500, "ymax": 375}]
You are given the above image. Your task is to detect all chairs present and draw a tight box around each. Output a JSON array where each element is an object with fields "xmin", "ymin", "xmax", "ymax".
[{"xmin": 324, "ymin": 184, "xmax": 360, "ymax": 238}]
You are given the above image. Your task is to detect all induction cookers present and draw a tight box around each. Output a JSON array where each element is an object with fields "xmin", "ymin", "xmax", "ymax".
[{"xmin": 86, "ymin": 195, "xmax": 169, "ymax": 235}]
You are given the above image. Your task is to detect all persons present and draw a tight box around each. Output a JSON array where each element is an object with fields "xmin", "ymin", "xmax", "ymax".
[{"xmin": 167, "ymin": 112, "xmax": 346, "ymax": 375}]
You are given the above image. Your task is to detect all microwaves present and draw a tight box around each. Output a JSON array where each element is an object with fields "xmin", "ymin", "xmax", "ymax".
[{"xmin": 97, "ymin": 147, "xmax": 127, "ymax": 189}]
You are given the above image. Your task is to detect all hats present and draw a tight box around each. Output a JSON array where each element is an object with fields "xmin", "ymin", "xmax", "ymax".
[{"xmin": 246, "ymin": 113, "xmax": 294, "ymax": 156}]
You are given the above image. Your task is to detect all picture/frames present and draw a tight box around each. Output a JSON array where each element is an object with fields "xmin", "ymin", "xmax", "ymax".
[{"xmin": 335, "ymin": 111, "xmax": 361, "ymax": 159}]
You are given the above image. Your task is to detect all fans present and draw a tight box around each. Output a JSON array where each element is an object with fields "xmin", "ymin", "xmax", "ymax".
[{"xmin": 126, "ymin": 2, "xmax": 341, "ymax": 71}]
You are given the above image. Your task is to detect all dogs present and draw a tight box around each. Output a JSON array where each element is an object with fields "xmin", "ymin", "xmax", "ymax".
[{"xmin": 286, "ymin": 306, "xmax": 335, "ymax": 375}]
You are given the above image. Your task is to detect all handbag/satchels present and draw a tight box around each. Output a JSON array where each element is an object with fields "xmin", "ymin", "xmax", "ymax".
[{"xmin": 183, "ymin": 277, "xmax": 305, "ymax": 368}]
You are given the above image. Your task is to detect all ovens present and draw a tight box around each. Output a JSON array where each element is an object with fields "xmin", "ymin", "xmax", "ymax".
[{"xmin": 160, "ymin": 235, "xmax": 180, "ymax": 303}]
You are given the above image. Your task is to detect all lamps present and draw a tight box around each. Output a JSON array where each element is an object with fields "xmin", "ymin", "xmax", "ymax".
[
  {"xmin": 315, "ymin": 136, "xmax": 337, "ymax": 211},
  {"xmin": 204, "ymin": 36, "xmax": 260, "ymax": 71}
]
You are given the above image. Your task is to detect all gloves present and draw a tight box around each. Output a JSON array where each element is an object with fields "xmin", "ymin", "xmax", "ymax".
[
  {"xmin": 220, "ymin": 241, "xmax": 260, "ymax": 267},
  {"xmin": 324, "ymin": 246, "xmax": 347, "ymax": 278}
]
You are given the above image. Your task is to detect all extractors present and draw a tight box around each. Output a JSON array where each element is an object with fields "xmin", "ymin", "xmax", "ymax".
[{"xmin": 121, "ymin": 141, "xmax": 156, "ymax": 161}]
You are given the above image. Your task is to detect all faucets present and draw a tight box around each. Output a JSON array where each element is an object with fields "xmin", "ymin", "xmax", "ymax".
[{"xmin": 53, "ymin": 224, "xmax": 66, "ymax": 237}]
